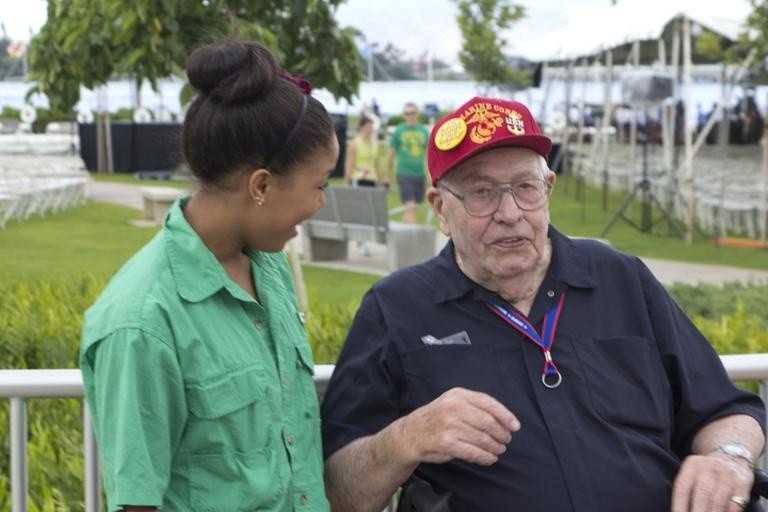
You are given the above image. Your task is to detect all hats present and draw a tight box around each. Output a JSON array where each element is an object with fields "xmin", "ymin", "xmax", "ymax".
[{"xmin": 428, "ymin": 96, "xmax": 552, "ymax": 188}]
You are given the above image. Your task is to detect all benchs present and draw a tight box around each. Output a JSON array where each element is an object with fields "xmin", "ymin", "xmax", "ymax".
[
  {"xmin": 137, "ymin": 185, "xmax": 188, "ymax": 219},
  {"xmin": 296, "ymin": 182, "xmax": 438, "ymax": 275}
]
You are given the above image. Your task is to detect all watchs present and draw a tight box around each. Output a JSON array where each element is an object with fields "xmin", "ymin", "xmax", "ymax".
[{"xmin": 710, "ymin": 439, "xmax": 755, "ymax": 470}]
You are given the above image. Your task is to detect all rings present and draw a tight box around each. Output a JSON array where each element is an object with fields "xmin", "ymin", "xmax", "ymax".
[{"xmin": 729, "ymin": 495, "xmax": 750, "ymax": 509}]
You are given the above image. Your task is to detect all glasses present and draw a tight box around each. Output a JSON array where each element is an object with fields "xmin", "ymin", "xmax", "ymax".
[
  {"xmin": 405, "ymin": 112, "xmax": 416, "ymax": 115},
  {"xmin": 437, "ymin": 178, "xmax": 548, "ymax": 217}
]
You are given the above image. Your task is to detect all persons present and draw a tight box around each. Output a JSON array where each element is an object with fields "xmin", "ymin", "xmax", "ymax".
[
  {"xmin": 343, "ymin": 118, "xmax": 384, "ymax": 187},
  {"xmin": 318, "ymin": 96, "xmax": 768, "ymax": 512},
  {"xmin": 360, "ymin": 103, "xmax": 381, "ymax": 169},
  {"xmin": 382, "ymin": 102, "xmax": 430, "ymax": 226},
  {"xmin": 81, "ymin": 41, "xmax": 335, "ymax": 512}
]
